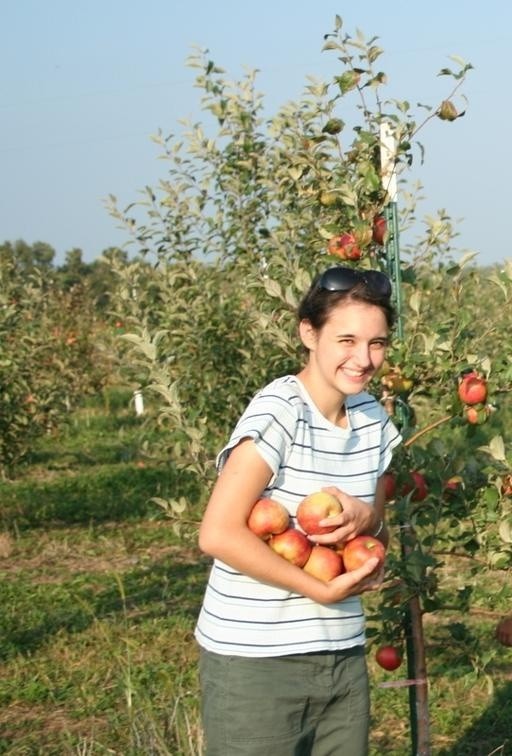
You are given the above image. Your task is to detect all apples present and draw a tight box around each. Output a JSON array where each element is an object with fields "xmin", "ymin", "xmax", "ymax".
[
  {"xmin": 376, "ymin": 646, "xmax": 402, "ymax": 670},
  {"xmin": 329, "ymin": 218, "xmax": 387, "ymax": 260},
  {"xmin": 266, "ymin": 528, "xmax": 313, "ymax": 568},
  {"xmin": 247, "ymin": 498, "xmax": 290, "ymax": 541},
  {"xmin": 459, "ymin": 369, "xmax": 488, "ymax": 424},
  {"xmin": 302, "ymin": 546, "xmax": 343, "ymax": 583},
  {"xmin": 502, "ymin": 475, "xmax": 512, "ymax": 495},
  {"xmin": 384, "ymin": 470, "xmax": 464, "ymax": 500},
  {"xmin": 497, "ymin": 619, "xmax": 512, "ymax": 646},
  {"xmin": 380, "ymin": 366, "xmax": 414, "ymax": 394},
  {"xmin": 343, "ymin": 535, "xmax": 385, "ymax": 573},
  {"xmin": 296, "ymin": 493, "xmax": 343, "ymax": 535}
]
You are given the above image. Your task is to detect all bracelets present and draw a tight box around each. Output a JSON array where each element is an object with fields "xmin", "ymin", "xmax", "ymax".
[{"xmin": 374, "ymin": 520, "xmax": 384, "ymax": 537}]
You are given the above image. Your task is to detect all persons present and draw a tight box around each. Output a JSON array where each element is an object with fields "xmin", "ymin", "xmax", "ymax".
[
  {"xmin": 194, "ymin": 268, "xmax": 404, "ymax": 756},
  {"xmin": 495, "ymin": 618, "xmax": 512, "ymax": 646}
]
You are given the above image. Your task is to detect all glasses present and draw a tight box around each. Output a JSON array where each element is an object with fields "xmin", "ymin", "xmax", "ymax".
[{"xmin": 305, "ymin": 268, "xmax": 391, "ymax": 304}]
